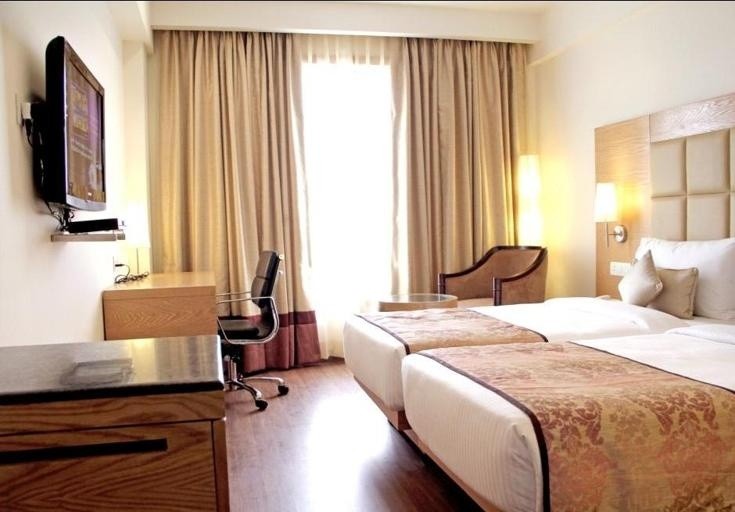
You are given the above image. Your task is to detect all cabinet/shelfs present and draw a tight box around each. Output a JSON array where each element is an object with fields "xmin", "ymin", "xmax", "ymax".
[{"xmin": 0, "ymin": 334, "xmax": 231, "ymax": 512}]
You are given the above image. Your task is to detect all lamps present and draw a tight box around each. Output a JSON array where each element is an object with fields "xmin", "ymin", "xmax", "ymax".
[{"xmin": 592, "ymin": 182, "xmax": 628, "ymax": 250}]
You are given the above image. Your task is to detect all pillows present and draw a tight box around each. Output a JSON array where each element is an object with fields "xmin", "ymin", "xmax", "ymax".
[{"xmin": 618, "ymin": 237, "xmax": 735, "ymax": 322}]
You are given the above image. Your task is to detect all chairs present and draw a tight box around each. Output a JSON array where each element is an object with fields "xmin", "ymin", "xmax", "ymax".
[
  {"xmin": 215, "ymin": 249, "xmax": 290, "ymax": 409},
  {"xmin": 438, "ymin": 244, "xmax": 549, "ymax": 307}
]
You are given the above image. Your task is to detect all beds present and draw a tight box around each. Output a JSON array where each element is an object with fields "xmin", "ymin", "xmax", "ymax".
[
  {"xmin": 342, "ymin": 294, "xmax": 726, "ymax": 430},
  {"xmin": 401, "ymin": 324, "xmax": 734, "ymax": 512}
]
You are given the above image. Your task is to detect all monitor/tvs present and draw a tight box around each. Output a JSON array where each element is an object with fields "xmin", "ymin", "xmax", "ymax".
[{"xmin": 44, "ymin": 36, "xmax": 107, "ymax": 211}]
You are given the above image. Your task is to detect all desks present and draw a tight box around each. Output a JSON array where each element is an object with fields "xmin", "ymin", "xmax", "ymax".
[
  {"xmin": 379, "ymin": 292, "xmax": 458, "ymax": 312},
  {"xmin": 102, "ymin": 270, "xmax": 218, "ymax": 343}
]
